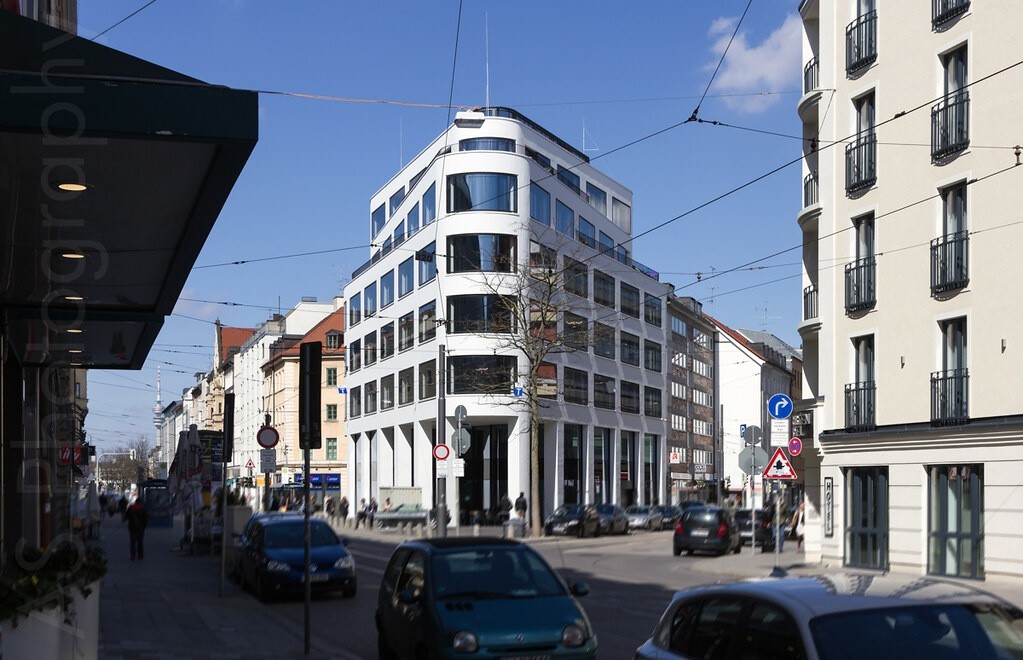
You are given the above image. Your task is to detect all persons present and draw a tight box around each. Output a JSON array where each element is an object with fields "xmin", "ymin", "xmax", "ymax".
[
  {"xmin": 98, "ymin": 493, "xmax": 108, "ymax": 520},
  {"xmin": 262, "ymin": 485, "xmax": 392, "ymax": 531},
  {"xmin": 731, "ymin": 494, "xmax": 742, "ymax": 507},
  {"xmin": 98, "ymin": 482, "xmax": 111, "ymax": 495},
  {"xmin": 763, "ymin": 491, "xmax": 804, "ymax": 554},
  {"xmin": 498, "ymin": 493, "xmax": 527, "ymax": 531},
  {"xmin": 118, "ymin": 495, "xmax": 128, "ymax": 522},
  {"xmin": 236, "ymin": 477, "xmax": 253, "ymax": 489},
  {"xmin": 108, "ymin": 498, "xmax": 117, "ymax": 520},
  {"xmin": 122, "ymin": 497, "xmax": 148, "ymax": 561}
]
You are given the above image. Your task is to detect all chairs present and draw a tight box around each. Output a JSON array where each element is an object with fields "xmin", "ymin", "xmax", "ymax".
[{"xmin": 480, "ymin": 556, "xmax": 523, "ymax": 593}]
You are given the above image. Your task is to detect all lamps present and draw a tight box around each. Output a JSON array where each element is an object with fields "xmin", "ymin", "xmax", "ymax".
[{"xmin": 55, "ymin": 160, "xmax": 87, "ymax": 366}]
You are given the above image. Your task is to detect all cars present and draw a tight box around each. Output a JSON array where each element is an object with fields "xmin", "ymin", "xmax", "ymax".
[
  {"xmin": 629, "ymin": 566, "xmax": 1023, "ymax": 660},
  {"xmin": 234, "ymin": 511, "xmax": 358, "ymax": 599},
  {"xmin": 374, "ymin": 535, "xmax": 601, "ymax": 660},
  {"xmin": 546, "ymin": 500, "xmax": 779, "ymax": 557}
]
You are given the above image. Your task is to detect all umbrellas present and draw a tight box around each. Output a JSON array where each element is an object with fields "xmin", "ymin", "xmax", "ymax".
[{"xmin": 167, "ymin": 424, "xmax": 204, "ymax": 555}]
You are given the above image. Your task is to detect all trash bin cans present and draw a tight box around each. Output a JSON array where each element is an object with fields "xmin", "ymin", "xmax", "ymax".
[
  {"xmin": 761, "ymin": 528, "xmax": 775, "ymax": 553},
  {"xmin": 504, "ymin": 520, "xmax": 525, "ymax": 540}
]
